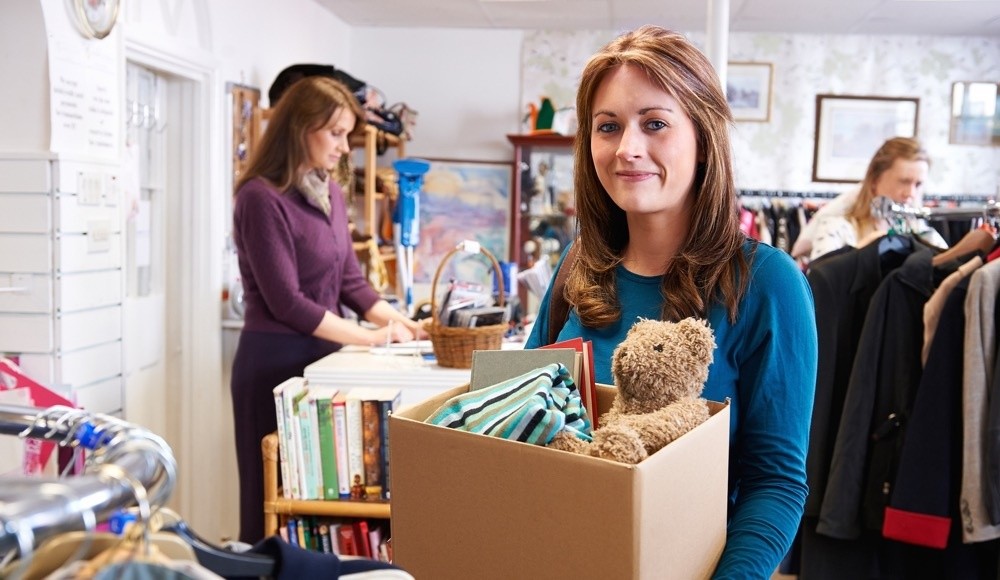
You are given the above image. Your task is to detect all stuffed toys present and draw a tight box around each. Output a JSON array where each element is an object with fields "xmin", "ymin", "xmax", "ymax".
[{"xmin": 544, "ymin": 316, "xmax": 718, "ymax": 465}]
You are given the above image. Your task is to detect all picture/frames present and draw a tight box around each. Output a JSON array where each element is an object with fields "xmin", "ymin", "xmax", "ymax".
[
  {"xmin": 813, "ymin": 95, "xmax": 918, "ymax": 182},
  {"xmin": 727, "ymin": 62, "xmax": 773, "ymax": 122}
]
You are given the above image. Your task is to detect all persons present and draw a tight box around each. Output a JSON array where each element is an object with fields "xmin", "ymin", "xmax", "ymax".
[
  {"xmin": 229, "ymin": 76, "xmax": 431, "ymax": 546},
  {"xmin": 791, "ymin": 137, "xmax": 930, "ymax": 271},
  {"xmin": 524, "ymin": 24, "xmax": 819, "ymax": 580}
]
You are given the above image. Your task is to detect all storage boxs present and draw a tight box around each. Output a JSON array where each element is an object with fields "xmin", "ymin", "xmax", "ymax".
[{"xmin": 392, "ymin": 385, "xmax": 730, "ymax": 580}]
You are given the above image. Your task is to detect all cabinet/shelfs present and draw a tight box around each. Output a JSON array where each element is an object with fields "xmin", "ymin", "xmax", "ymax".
[{"xmin": 252, "ymin": 107, "xmax": 404, "ymax": 292}]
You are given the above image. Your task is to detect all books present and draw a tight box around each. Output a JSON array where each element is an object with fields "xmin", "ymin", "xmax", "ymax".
[
  {"xmin": 441, "ymin": 256, "xmax": 552, "ymax": 329},
  {"xmin": 469, "ymin": 348, "xmax": 583, "ymax": 392},
  {"xmin": 272, "ymin": 376, "xmax": 401, "ymax": 565},
  {"xmin": 535, "ymin": 337, "xmax": 599, "ymax": 429}
]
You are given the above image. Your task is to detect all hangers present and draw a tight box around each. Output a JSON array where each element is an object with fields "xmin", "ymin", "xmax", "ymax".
[
  {"xmin": 870, "ymin": 201, "xmax": 1000, "ymax": 266},
  {"xmin": 0, "ymin": 406, "xmax": 274, "ymax": 580}
]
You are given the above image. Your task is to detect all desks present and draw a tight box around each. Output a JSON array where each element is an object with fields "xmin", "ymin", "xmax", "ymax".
[{"xmin": 305, "ymin": 341, "xmax": 524, "ymax": 400}]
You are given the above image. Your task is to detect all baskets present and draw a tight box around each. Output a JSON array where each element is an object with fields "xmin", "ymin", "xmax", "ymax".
[{"xmin": 422, "ymin": 247, "xmax": 509, "ymax": 368}]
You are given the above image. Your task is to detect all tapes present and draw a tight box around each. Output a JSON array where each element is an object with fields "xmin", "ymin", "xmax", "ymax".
[{"xmin": 415, "ymin": 299, "xmax": 438, "ymax": 318}]
